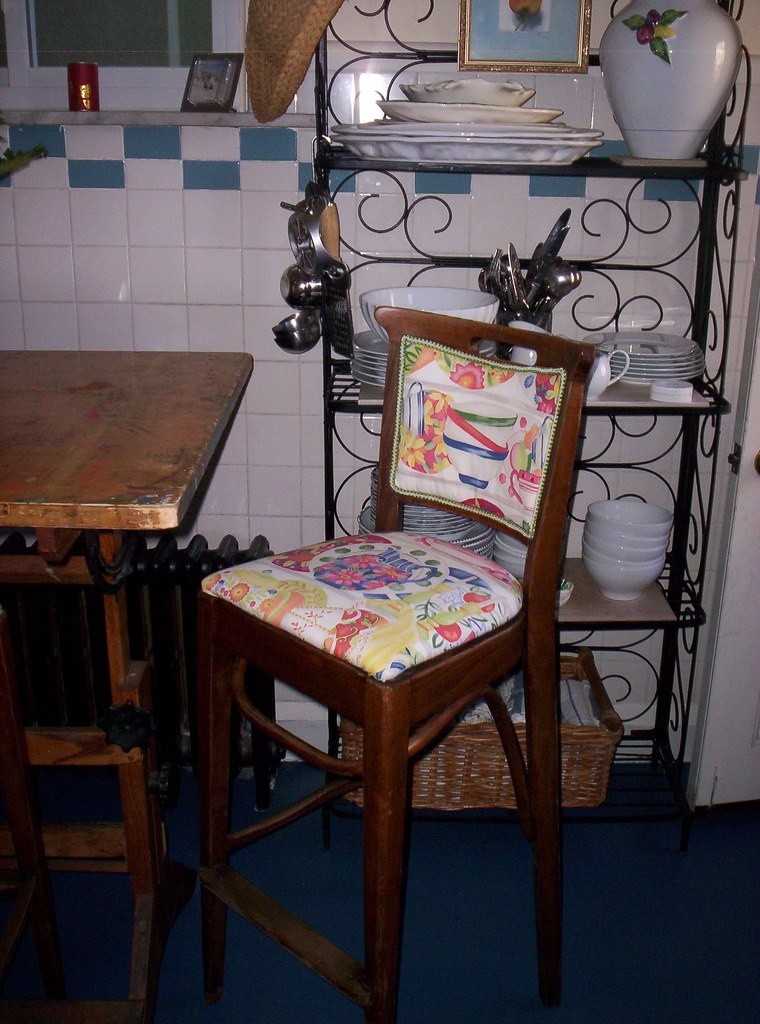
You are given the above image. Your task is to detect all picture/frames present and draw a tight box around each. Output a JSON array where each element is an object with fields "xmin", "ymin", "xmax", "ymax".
[
  {"xmin": 457, "ymin": 0, "xmax": 592, "ymax": 74},
  {"xmin": 179, "ymin": 52, "xmax": 245, "ymax": 113}
]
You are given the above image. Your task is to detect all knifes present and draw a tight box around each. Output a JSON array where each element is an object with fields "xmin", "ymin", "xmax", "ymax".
[{"xmin": 508, "ymin": 208, "xmax": 571, "ymax": 304}]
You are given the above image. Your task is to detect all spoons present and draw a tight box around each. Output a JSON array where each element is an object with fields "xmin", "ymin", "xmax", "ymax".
[{"xmin": 529, "ymin": 266, "xmax": 567, "ymax": 320}]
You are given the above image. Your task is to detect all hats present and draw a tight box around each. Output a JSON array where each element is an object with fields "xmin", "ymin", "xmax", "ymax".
[{"xmin": 245, "ymin": 0, "xmax": 345, "ymax": 122}]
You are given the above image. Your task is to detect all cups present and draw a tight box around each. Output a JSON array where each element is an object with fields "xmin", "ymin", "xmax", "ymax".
[{"xmin": 508, "ymin": 320, "xmax": 553, "ymax": 366}]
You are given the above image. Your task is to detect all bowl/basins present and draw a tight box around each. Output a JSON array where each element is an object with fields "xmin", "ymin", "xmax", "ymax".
[
  {"xmin": 581, "ymin": 500, "xmax": 674, "ymax": 601},
  {"xmin": 359, "ymin": 287, "xmax": 502, "ymax": 344}
]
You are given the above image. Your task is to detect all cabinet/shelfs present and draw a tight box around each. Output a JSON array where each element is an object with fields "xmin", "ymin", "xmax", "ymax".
[{"xmin": 314, "ymin": 0, "xmax": 752, "ymax": 850}]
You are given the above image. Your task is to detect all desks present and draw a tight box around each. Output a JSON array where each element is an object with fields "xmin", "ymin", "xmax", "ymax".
[{"xmin": 0, "ymin": 349, "xmax": 254, "ymax": 1024}]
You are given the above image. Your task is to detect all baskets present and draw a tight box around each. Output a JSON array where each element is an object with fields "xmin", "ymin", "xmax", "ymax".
[{"xmin": 345, "ymin": 645, "xmax": 624, "ymax": 811}]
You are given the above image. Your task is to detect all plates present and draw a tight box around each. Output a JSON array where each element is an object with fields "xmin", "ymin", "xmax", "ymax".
[
  {"xmin": 349, "ymin": 331, "xmax": 498, "ymax": 389},
  {"xmin": 357, "ymin": 464, "xmax": 528, "ymax": 579},
  {"xmin": 329, "ymin": 78, "xmax": 604, "ymax": 166},
  {"xmin": 583, "ymin": 331, "xmax": 707, "ymax": 383}
]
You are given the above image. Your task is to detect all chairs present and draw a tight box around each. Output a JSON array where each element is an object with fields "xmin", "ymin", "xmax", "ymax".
[{"xmin": 197, "ymin": 304, "xmax": 601, "ymax": 1024}]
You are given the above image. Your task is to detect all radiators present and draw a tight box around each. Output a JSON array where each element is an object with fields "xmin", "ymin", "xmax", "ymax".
[{"xmin": 0, "ymin": 532, "xmax": 287, "ymax": 810}]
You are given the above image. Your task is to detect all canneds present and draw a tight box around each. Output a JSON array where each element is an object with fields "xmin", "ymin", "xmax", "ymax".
[{"xmin": 66, "ymin": 61, "xmax": 100, "ymax": 112}]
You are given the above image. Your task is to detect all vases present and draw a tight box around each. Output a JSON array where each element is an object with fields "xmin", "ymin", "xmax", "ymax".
[{"xmin": 597, "ymin": 0, "xmax": 742, "ymax": 159}]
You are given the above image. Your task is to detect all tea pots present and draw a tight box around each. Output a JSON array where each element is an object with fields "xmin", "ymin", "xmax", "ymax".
[{"xmin": 582, "ymin": 349, "xmax": 629, "ymax": 400}]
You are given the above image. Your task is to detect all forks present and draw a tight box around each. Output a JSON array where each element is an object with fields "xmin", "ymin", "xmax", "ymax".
[{"xmin": 490, "ymin": 248, "xmax": 504, "ymax": 285}]
